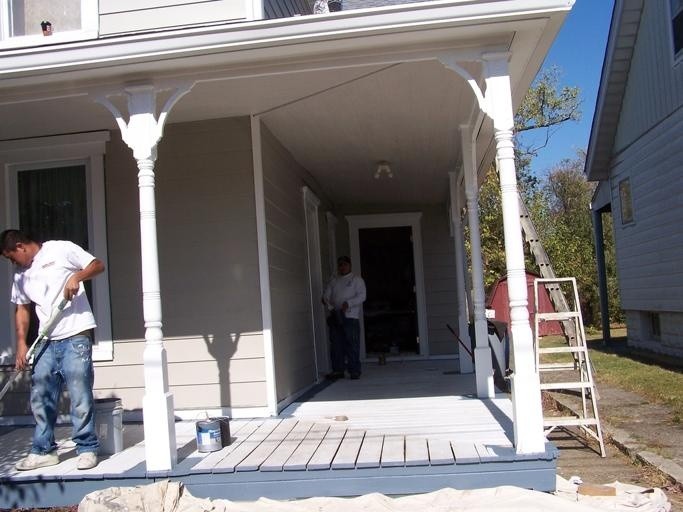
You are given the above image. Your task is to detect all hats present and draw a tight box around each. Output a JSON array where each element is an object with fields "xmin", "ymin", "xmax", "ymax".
[{"xmin": 336, "ymin": 256, "xmax": 351, "ymax": 265}]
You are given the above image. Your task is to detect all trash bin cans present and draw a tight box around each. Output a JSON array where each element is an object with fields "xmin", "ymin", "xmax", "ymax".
[{"xmin": 468, "ymin": 320, "xmax": 510, "ymax": 394}]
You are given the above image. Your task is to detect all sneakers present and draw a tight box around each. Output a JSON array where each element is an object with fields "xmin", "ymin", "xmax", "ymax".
[
  {"xmin": 351, "ymin": 371, "xmax": 360, "ymax": 380},
  {"xmin": 15, "ymin": 450, "xmax": 60, "ymax": 471},
  {"xmin": 77, "ymin": 451, "xmax": 97, "ymax": 470},
  {"xmin": 325, "ymin": 371, "xmax": 344, "ymax": 380}
]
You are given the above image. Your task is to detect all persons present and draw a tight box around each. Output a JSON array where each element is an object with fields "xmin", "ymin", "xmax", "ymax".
[
  {"xmin": 0, "ymin": 229, "xmax": 105, "ymax": 471},
  {"xmin": 322, "ymin": 256, "xmax": 366, "ymax": 379}
]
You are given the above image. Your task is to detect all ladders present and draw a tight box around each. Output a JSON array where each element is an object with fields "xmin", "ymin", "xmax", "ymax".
[
  {"xmin": 492, "ymin": 157, "xmax": 602, "ymax": 402},
  {"xmin": 533, "ymin": 277, "xmax": 605, "ymax": 458}
]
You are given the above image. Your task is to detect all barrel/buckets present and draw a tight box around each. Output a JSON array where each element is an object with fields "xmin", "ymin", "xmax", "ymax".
[
  {"xmin": 195, "ymin": 416, "xmax": 232, "ymax": 453},
  {"xmin": 93, "ymin": 397, "xmax": 126, "ymax": 456}
]
